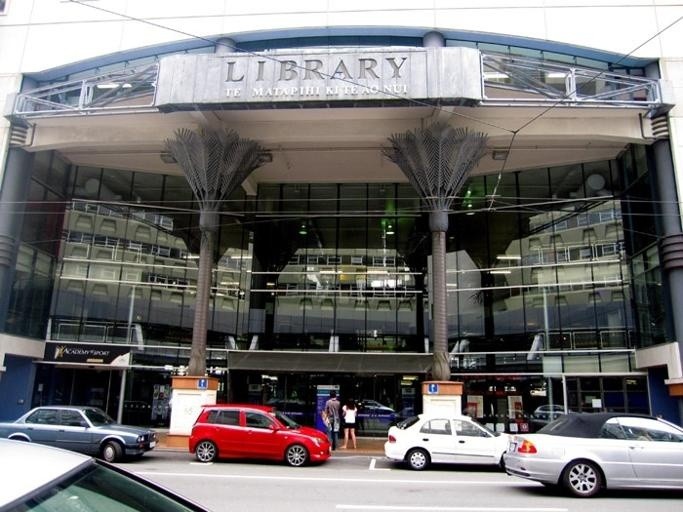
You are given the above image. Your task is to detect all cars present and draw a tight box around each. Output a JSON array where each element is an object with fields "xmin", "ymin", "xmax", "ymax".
[
  {"xmin": 0, "ymin": 405, "xmax": 160, "ymax": 463},
  {"xmin": 357, "ymin": 399, "xmax": 395, "ymax": 415},
  {"xmin": 189, "ymin": 404, "xmax": 333, "ymax": 468},
  {"xmin": 535, "ymin": 405, "xmax": 575, "ymax": 421},
  {"xmin": 503, "ymin": 412, "xmax": 683, "ymax": 496},
  {"xmin": 385, "ymin": 412, "xmax": 517, "ymax": 471},
  {"xmin": 0, "ymin": 436, "xmax": 211, "ymax": 512}
]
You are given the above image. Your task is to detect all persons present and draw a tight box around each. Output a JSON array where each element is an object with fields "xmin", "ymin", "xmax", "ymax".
[
  {"xmin": 338, "ymin": 396, "xmax": 358, "ymax": 449},
  {"xmin": 152, "ymin": 392, "xmax": 167, "ymax": 428},
  {"xmin": 324, "ymin": 389, "xmax": 340, "ymax": 450}
]
